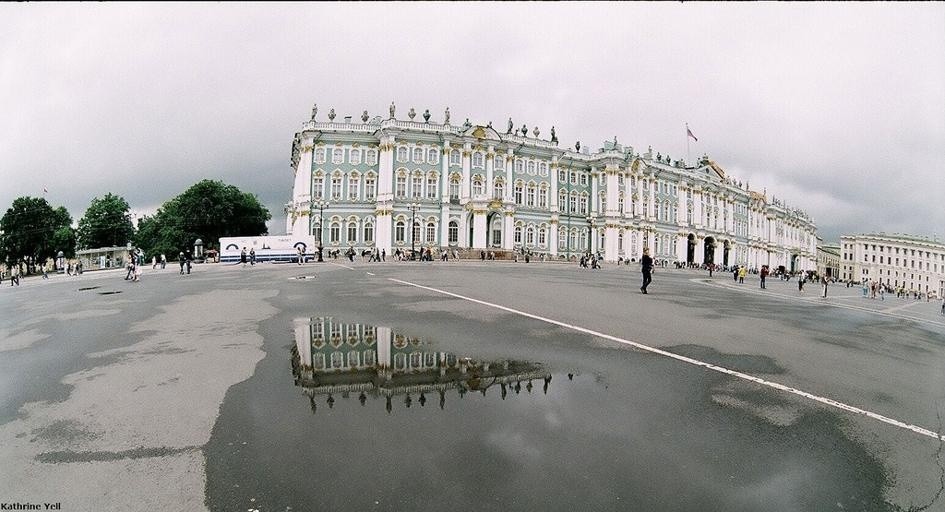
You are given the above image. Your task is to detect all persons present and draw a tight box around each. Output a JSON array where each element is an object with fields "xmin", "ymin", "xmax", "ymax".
[
  {"xmin": 487, "ymin": 121, "xmax": 492, "ymax": 128},
  {"xmin": 302, "ymin": 370, "xmax": 575, "ymax": 415},
  {"xmin": 666, "ymin": 154, "xmax": 670, "ymax": 166},
  {"xmin": 657, "ymin": 152, "xmax": 661, "ymax": 161},
  {"xmin": 575, "ymin": 141, "xmax": 580, "ymax": 153},
  {"xmin": 389, "ymin": 101, "xmax": 396, "ymax": 119},
  {"xmin": 523, "ymin": 125, "xmax": 527, "ymax": 136},
  {"xmin": 508, "ymin": 118, "xmax": 513, "ymax": 133},
  {"xmin": 462, "ymin": 118, "xmax": 471, "ymax": 127},
  {"xmin": 311, "ymin": 103, "xmax": 317, "ymax": 119},
  {"xmin": 360, "ymin": 110, "xmax": 370, "ymax": 124},
  {"xmin": 408, "ymin": 107, "xmax": 416, "ymax": 120},
  {"xmin": 613, "ymin": 135, "xmax": 617, "ymax": 147},
  {"xmin": 680, "ymin": 152, "xmax": 751, "ymax": 192},
  {"xmin": 551, "ymin": 125, "xmax": 556, "ymax": 142},
  {"xmin": 328, "ymin": 107, "xmax": 336, "ymax": 122},
  {"xmin": 423, "ymin": 109, "xmax": 430, "ymax": 121},
  {"xmin": 445, "ymin": 106, "xmax": 451, "ymax": 124},
  {"xmin": 648, "ymin": 144, "xmax": 654, "ymax": 159},
  {"xmin": 533, "ymin": 127, "xmax": 539, "ymax": 138}
]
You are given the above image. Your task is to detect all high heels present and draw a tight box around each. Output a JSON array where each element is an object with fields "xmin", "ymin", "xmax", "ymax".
[{"xmin": 640, "ymin": 287, "xmax": 647, "ymax": 294}]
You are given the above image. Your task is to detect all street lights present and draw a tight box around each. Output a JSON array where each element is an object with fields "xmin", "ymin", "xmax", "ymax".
[
  {"xmin": 586, "ymin": 216, "xmax": 596, "ymax": 255},
  {"xmin": 314, "ymin": 201, "xmax": 330, "ymax": 261},
  {"xmin": 406, "ymin": 202, "xmax": 421, "ymax": 262},
  {"xmin": 643, "ymin": 224, "xmax": 652, "ymax": 248}
]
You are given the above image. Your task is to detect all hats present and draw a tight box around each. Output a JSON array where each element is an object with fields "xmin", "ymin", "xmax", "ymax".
[{"xmin": 643, "ymin": 247, "xmax": 649, "ymax": 252}]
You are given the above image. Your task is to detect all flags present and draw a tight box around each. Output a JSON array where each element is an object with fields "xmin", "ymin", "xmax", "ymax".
[{"xmin": 687, "ymin": 127, "xmax": 698, "ymax": 142}]
